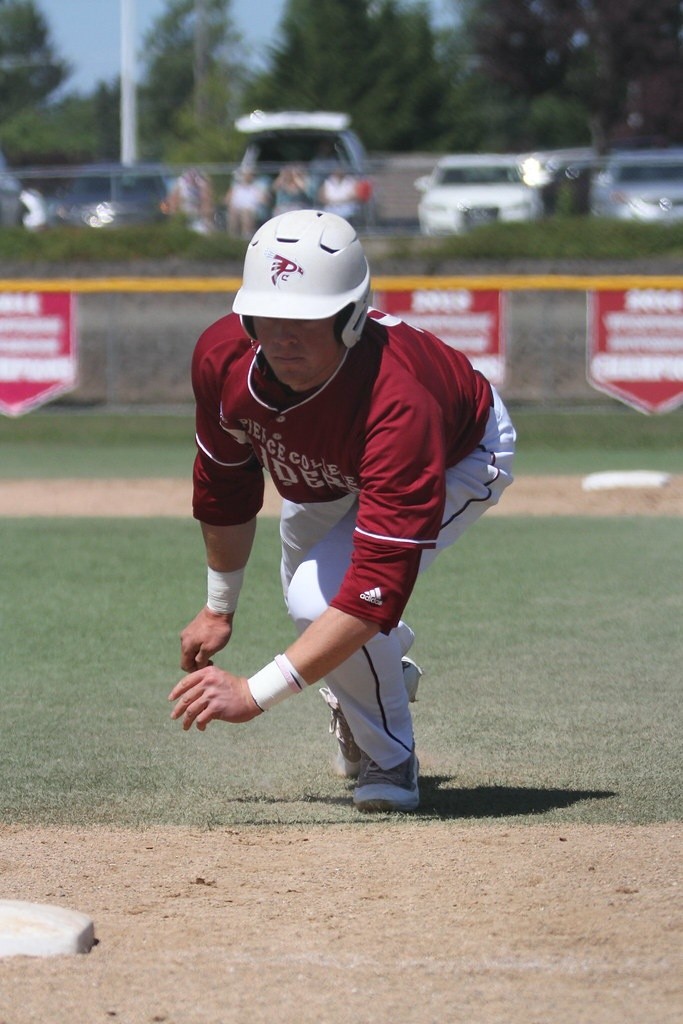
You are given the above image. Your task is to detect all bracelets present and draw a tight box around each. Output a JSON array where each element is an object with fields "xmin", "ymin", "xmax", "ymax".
[
  {"xmin": 206, "ymin": 568, "xmax": 242, "ymax": 614},
  {"xmin": 274, "ymin": 654, "xmax": 302, "ymax": 694},
  {"xmin": 247, "ymin": 653, "xmax": 309, "ymax": 712}
]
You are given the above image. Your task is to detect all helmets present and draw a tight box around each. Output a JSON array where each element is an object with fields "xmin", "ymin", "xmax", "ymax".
[{"xmin": 232, "ymin": 208, "xmax": 372, "ymax": 345}]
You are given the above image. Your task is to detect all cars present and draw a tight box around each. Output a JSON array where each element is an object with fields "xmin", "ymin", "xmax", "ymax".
[
  {"xmin": 588, "ymin": 147, "xmax": 683, "ymax": 222},
  {"xmin": 413, "ymin": 153, "xmax": 545, "ymax": 237},
  {"xmin": 48, "ymin": 163, "xmax": 173, "ymax": 226}
]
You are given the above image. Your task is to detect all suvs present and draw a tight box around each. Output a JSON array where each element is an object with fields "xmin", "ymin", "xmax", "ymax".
[{"xmin": 226, "ymin": 112, "xmax": 384, "ymax": 233}]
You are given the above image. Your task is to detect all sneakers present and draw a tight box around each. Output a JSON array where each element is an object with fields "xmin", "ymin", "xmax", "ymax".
[
  {"xmin": 319, "ymin": 654, "xmax": 422, "ymax": 778},
  {"xmin": 354, "ymin": 738, "xmax": 420, "ymax": 811}
]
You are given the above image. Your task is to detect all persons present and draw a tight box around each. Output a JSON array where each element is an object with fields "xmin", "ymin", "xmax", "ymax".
[
  {"xmin": 167, "ymin": 209, "xmax": 517, "ymax": 812},
  {"xmin": 161, "ymin": 164, "xmax": 359, "ymax": 237}
]
